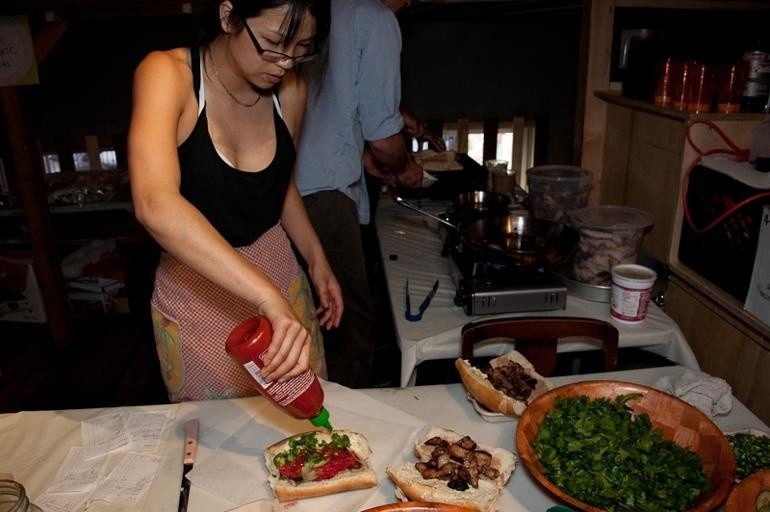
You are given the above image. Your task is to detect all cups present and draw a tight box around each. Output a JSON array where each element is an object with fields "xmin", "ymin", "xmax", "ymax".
[
  {"xmin": 572, "ymin": 214, "xmax": 643, "ymax": 270},
  {"xmin": 609, "ymin": 263, "xmax": 658, "ymax": 325},
  {"xmin": 653, "ymin": 56, "xmax": 745, "ymax": 113},
  {"xmin": 527, "ymin": 163, "xmax": 593, "ymax": 218}
]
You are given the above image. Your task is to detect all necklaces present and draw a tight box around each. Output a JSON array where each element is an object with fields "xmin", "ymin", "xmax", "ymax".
[{"xmin": 208, "ymin": 43, "xmax": 263, "ymax": 107}]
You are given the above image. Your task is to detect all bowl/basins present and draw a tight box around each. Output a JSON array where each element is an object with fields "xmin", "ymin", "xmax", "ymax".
[
  {"xmin": 518, "ymin": 379, "xmax": 736, "ymax": 510},
  {"xmin": 565, "ymin": 275, "xmax": 614, "ymax": 307}
]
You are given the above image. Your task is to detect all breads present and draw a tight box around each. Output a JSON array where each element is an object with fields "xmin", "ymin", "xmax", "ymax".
[
  {"xmin": 454, "ymin": 349, "xmax": 549, "ymax": 417},
  {"xmin": 386, "ymin": 425, "xmax": 518, "ymax": 512},
  {"xmin": 264, "ymin": 429, "xmax": 378, "ymax": 503}
]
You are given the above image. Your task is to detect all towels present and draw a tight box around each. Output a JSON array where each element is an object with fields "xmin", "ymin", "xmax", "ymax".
[{"xmin": 653, "ymin": 367, "xmax": 734, "ymax": 421}]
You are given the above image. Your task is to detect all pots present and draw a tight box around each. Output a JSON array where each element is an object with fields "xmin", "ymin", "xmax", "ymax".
[{"xmin": 393, "ymin": 151, "xmax": 564, "ymax": 267}]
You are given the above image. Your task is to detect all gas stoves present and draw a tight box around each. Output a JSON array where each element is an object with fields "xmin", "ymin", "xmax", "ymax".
[{"xmin": 435, "ymin": 216, "xmax": 569, "ymax": 316}]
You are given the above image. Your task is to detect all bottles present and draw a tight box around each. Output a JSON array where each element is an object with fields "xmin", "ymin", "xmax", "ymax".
[{"xmin": 225, "ymin": 315, "xmax": 338, "ymax": 434}]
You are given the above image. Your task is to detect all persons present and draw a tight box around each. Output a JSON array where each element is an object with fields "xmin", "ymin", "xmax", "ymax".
[
  {"xmin": 126, "ymin": 0, "xmax": 344, "ymax": 404},
  {"xmin": 293, "ymin": 0, "xmax": 423, "ymax": 390},
  {"xmin": 400, "ymin": 104, "xmax": 446, "ymax": 152}
]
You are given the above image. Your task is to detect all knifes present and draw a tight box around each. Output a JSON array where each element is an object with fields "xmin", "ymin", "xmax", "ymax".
[{"xmin": 178, "ymin": 418, "xmax": 200, "ymax": 511}]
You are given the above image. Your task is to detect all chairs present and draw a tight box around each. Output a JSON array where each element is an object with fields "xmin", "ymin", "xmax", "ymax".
[{"xmin": 460, "ymin": 315, "xmax": 620, "ymax": 384}]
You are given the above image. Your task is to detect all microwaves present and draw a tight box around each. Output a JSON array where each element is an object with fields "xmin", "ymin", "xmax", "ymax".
[{"xmin": 678, "ymin": 150, "xmax": 770, "ymax": 332}]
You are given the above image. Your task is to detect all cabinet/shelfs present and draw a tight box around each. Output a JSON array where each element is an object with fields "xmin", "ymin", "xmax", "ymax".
[
  {"xmin": 593, "ymin": 88, "xmax": 770, "ymax": 268},
  {"xmin": 0, "ymin": 0, "xmax": 150, "ymax": 407},
  {"xmin": 656, "ymin": 270, "xmax": 770, "ymax": 426}
]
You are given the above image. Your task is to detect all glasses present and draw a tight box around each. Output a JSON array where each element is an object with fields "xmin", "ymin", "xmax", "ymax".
[{"xmin": 239, "ymin": 15, "xmax": 323, "ymax": 69}]
